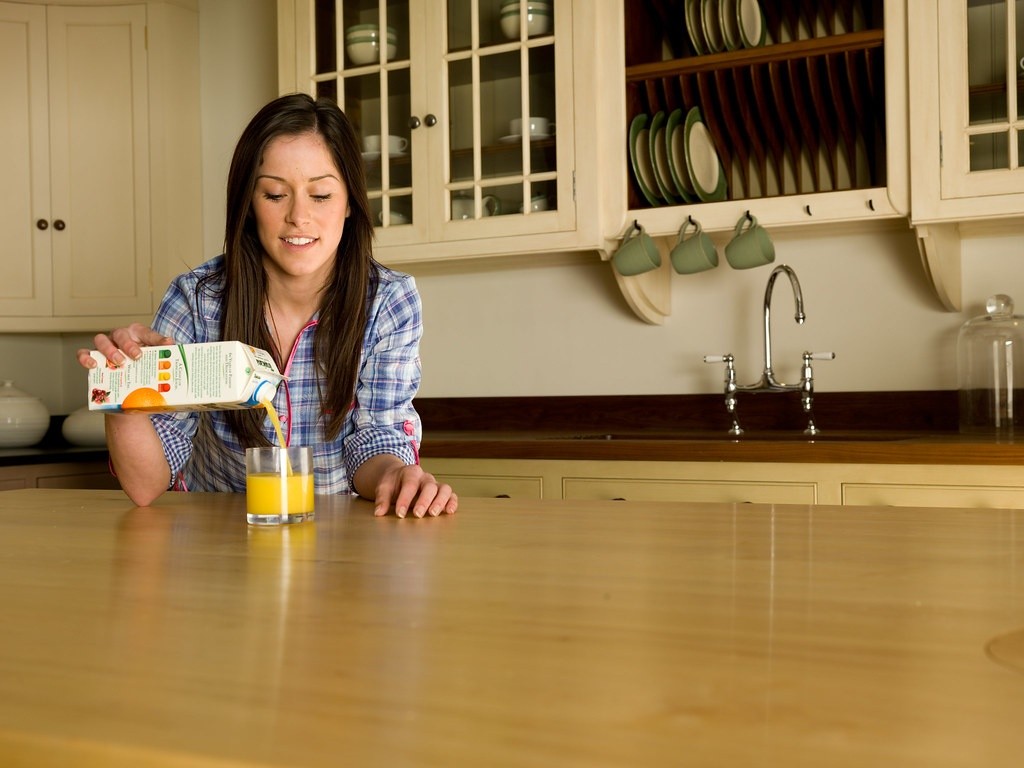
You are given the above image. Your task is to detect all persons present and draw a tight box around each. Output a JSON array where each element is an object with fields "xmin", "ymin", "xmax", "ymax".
[{"xmin": 77, "ymin": 91, "xmax": 460, "ymax": 521}]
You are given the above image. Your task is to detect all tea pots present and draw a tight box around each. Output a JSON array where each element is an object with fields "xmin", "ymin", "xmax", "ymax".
[{"xmin": 451, "ymin": 195, "xmax": 501, "ymax": 221}]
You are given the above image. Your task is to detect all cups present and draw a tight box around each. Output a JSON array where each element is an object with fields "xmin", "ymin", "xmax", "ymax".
[
  {"xmin": 379, "ymin": 210, "xmax": 410, "ymax": 224},
  {"xmin": 509, "ymin": 117, "xmax": 556, "ymax": 135},
  {"xmin": 246, "ymin": 446, "xmax": 315, "ymax": 525},
  {"xmin": 669, "ymin": 220, "xmax": 719, "ymax": 274},
  {"xmin": 363, "ymin": 135, "xmax": 408, "ymax": 151},
  {"xmin": 725, "ymin": 215, "xmax": 774, "ymax": 271},
  {"xmin": 613, "ymin": 224, "xmax": 661, "ymax": 276}
]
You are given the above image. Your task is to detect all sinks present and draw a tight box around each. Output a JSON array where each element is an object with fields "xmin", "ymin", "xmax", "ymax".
[{"xmin": 534, "ymin": 432, "xmax": 928, "ymax": 442}]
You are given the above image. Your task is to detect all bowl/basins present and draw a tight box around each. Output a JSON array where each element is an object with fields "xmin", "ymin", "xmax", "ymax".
[
  {"xmin": 348, "ymin": 22, "xmax": 395, "ymax": 65},
  {"xmin": 519, "ymin": 195, "xmax": 548, "ymax": 212},
  {"xmin": 0, "ymin": 379, "xmax": 49, "ymax": 447},
  {"xmin": 499, "ymin": 0, "xmax": 551, "ymax": 39},
  {"xmin": 62, "ymin": 405, "xmax": 107, "ymax": 447}
]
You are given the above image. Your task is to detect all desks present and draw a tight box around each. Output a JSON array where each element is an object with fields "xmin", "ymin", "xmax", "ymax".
[{"xmin": 0, "ymin": 485, "xmax": 1024, "ymax": 768}]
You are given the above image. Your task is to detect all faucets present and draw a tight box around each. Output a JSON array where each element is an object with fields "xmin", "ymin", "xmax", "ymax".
[{"xmin": 701, "ymin": 264, "xmax": 836, "ymax": 435}]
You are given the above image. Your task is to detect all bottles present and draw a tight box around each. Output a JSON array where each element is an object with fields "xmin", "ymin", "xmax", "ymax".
[{"xmin": 957, "ymin": 295, "xmax": 1024, "ymax": 441}]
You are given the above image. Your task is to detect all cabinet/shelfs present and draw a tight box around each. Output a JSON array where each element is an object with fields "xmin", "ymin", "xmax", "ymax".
[
  {"xmin": 279, "ymin": 0, "xmax": 1024, "ymax": 507},
  {"xmin": 0, "ymin": 0, "xmax": 201, "ymax": 333}
]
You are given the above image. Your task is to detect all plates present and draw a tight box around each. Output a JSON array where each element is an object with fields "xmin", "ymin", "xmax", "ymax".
[
  {"xmin": 498, "ymin": 135, "xmax": 550, "ymax": 143},
  {"xmin": 360, "ymin": 153, "xmax": 406, "ymax": 160},
  {"xmin": 625, "ymin": 0, "xmax": 765, "ymax": 206}
]
